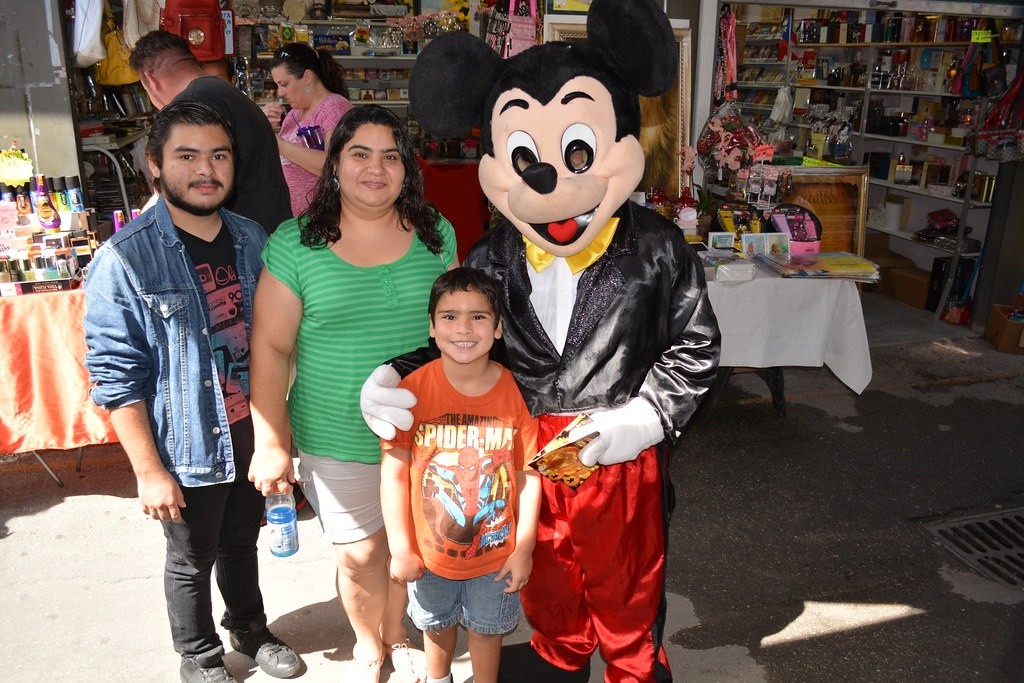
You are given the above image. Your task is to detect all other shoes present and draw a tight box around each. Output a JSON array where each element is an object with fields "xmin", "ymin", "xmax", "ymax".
[
  {"xmin": 349, "ymin": 642, "xmax": 384, "ymax": 683},
  {"xmin": 385, "ymin": 635, "xmax": 427, "ymax": 683}
]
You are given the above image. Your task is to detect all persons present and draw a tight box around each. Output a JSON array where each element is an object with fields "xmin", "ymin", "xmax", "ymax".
[
  {"xmin": 247, "ymin": 103, "xmax": 461, "ymax": 683},
  {"xmin": 126, "ymin": 32, "xmax": 308, "ymax": 529},
  {"xmin": 199, "ymin": 58, "xmax": 237, "ymax": 86},
  {"xmin": 79, "ymin": 99, "xmax": 301, "ymax": 683},
  {"xmin": 360, "ymin": 0, "xmax": 722, "ymax": 683},
  {"xmin": 380, "ymin": 268, "xmax": 542, "ymax": 683},
  {"xmin": 261, "ymin": 43, "xmax": 352, "ymax": 217}
]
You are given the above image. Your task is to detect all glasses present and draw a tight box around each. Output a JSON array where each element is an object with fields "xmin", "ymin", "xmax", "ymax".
[{"xmin": 273, "ymin": 47, "xmax": 294, "ymax": 58}]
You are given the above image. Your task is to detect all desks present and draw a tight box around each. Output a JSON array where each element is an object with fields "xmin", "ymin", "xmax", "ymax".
[
  {"xmin": 0, "ymin": 289, "xmax": 120, "ymax": 490},
  {"xmin": 705, "ymin": 258, "xmax": 872, "ymax": 422}
]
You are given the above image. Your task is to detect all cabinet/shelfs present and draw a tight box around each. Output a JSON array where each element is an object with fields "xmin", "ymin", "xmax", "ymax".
[
  {"xmin": 782, "ymin": 0, "xmax": 1024, "ymax": 322},
  {"xmin": 61, "ymin": 0, "xmax": 428, "ymax": 242},
  {"xmin": 738, "ymin": 20, "xmax": 800, "ymax": 145}
]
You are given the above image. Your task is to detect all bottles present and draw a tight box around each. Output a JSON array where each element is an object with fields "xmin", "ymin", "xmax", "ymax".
[
  {"xmin": 673, "ymin": 187, "xmax": 699, "ymax": 221},
  {"xmin": 265, "ymin": 486, "xmax": 299, "ymax": 556},
  {"xmin": 647, "ymin": 186, "xmax": 673, "ymax": 220}
]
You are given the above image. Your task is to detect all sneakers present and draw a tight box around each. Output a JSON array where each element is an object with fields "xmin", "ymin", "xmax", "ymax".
[
  {"xmin": 229, "ymin": 613, "xmax": 301, "ymax": 677},
  {"xmin": 179, "ymin": 645, "xmax": 237, "ymax": 683}
]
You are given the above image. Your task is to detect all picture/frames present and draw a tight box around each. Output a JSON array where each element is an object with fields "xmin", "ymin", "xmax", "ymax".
[
  {"xmin": 540, "ymin": 13, "xmax": 693, "ymax": 203},
  {"xmin": 325, "ymin": 0, "xmax": 421, "ymax": 55}
]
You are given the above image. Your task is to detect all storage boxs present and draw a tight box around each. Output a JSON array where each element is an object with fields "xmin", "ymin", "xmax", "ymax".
[
  {"xmin": 361, "ymin": 90, "xmax": 374, "ymax": 100},
  {"xmin": 893, "ymin": 164, "xmax": 913, "ymax": 185},
  {"xmin": 313, "ymin": 33, "xmax": 351, "ymax": 56},
  {"xmin": 885, "ymin": 193, "xmax": 912, "ymax": 228},
  {"xmin": 0, "ymin": 207, "xmax": 113, "ymax": 297},
  {"xmin": 868, "ymin": 256, "xmax": 916, "ymax": 295},
  {"xmin": 79, "ymin": 121, "xmax": 104, "ymax": 138},
  {"xmin": 886, "ymin": 267, "xmax": 932, "ymax": 310},
  {"xmin": 374, "ymin": 90, "xmax": 387, "ymax": 100},
  {"xmin": 736, "ymin": 24, "xmax": 747, "ymax": 65},
  {"xmin": 81, "ymin": 134, "xmax": 116, "ymax": 144},
  {"xmin": 920, "ymin": 161, "xmax": 950, "ymax": 189},
  {"xmin": 984, "ymin": 304, "xmax": 1024, "ymax": 355},
  {"xmin": 887, "ymin": 159, "xmax": 899, "ymax": 183},
  {"xmin": 400, "ymin": 89, "xmax": 409, "ymax": 99},
  {"xmin": 348, "ymin": 88, "xmax": 361, "ymax": 100},
  {"xmin": 869, "ymin": 151, "xmax": 896, "ymax": 180},
  {"xmin": 924, "ymin": 257, "xmax": 975, "ymax": 313},
  {"xmin": 910, "ymin": 160, "xmax": 924, "ymax": 185},
  {"xmin": 387, "ymin": 88, "xmax": 401, "ymax": 100},
  {"xmin": 927, "ymin": 126, "xmax": 971, "ymax": 146}
]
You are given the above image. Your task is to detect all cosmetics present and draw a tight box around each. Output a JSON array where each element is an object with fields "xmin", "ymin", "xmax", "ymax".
[{"xmin": 0, "ymin": 252, "xmax": 70, "ymax": 283}]
